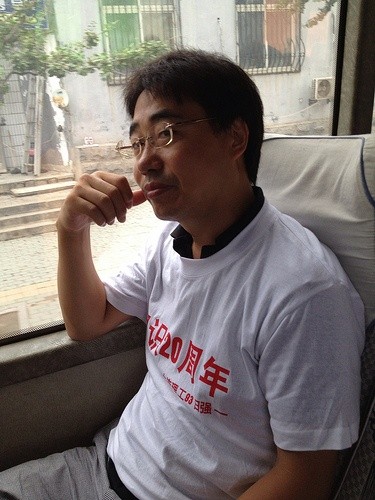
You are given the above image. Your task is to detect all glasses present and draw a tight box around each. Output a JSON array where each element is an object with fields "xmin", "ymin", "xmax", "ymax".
[{"xmin": 114, "ymin": 117, "xmax": 207, "ymax": 162}]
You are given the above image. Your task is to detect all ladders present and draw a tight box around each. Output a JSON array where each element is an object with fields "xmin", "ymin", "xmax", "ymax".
[{"xmin": 21, "ymin": 69, "xmax": 43, "ymax": 176}]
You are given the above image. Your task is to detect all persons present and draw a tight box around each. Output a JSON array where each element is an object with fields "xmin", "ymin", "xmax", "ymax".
[{"xmin": 0, "ymin": 49, "xmax": 365, "ymax": 500}]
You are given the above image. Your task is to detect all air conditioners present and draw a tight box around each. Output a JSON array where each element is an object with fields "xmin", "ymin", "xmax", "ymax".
[{"xmin": 311, "ymin": 78, "xmax": 335, "ymax": 102}]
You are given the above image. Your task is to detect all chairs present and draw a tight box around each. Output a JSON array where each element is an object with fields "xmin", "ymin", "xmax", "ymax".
[{"xmin": 256, "ymin": 133, "xmax": 375, "ymax": 500}]
